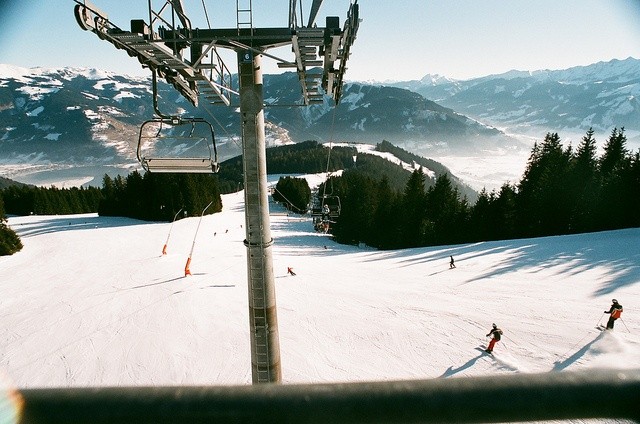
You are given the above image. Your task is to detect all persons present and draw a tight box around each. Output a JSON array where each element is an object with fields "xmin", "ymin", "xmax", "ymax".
[
  {"xmin": 484, "ymin": 322, "xmax": 503, "ymax": 353},
  {"xmin": 287, "ymin": 266, "xmax": 296, "ymax": 276},
  {"xmin": 604, "ymin": 299, "xmax": 623, "ymax": 330},
  {"xmin": 316, "ymin": 221, "xmax": 329, "ymax": 233},
  {"xmin": 321, "ymin": 204, "xmax": 331, "ymax": 220},
  {"xmin": 449, "ymin": 256, "xmax": 456, "ymax": 269}
]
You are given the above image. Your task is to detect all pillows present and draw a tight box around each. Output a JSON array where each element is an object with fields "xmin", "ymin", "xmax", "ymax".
[
  {"xmin": 612, "ymin": 299, "xmax": 617, "ymax": 303},
  {"xmin": 493, "ymin": 323, "xmax": 496, "ymax": 328}
]
